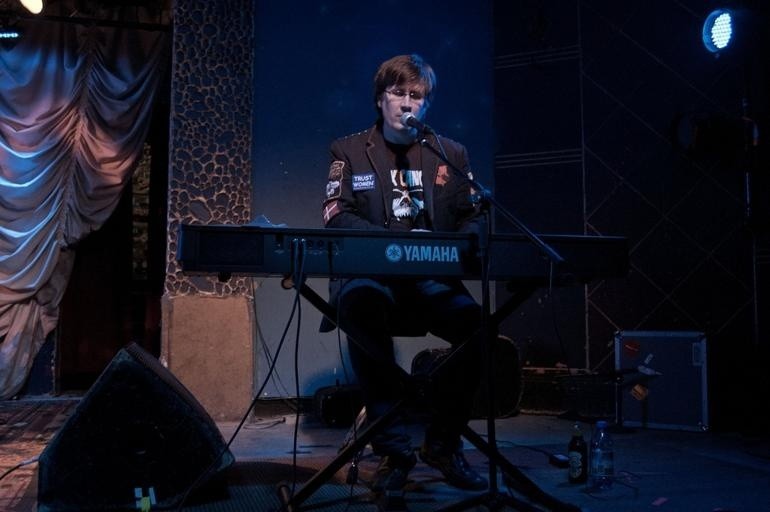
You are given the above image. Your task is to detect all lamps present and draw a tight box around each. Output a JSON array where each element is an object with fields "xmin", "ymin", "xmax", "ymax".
[{"xmin": 702, "ymin": 7, "xmax": 739, "ymax": 56}]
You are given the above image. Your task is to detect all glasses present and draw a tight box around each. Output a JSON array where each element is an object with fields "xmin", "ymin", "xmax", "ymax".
[{"xmin": 384, "ymin": 87, "xmax": 429, "ymax": 101}]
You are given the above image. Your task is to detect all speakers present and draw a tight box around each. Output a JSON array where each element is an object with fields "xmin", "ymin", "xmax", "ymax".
[{"xmin": 36, "ymin": 343, "xmax": 248, "ymax": 509}]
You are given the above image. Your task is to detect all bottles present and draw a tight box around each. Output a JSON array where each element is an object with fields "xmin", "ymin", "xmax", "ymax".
[
  {"xmin": 587, "ymin": 421, "xmax": 616, "ymax": 500},
  {"xmin": 565, "ymin": 425, "xmax": 588, "ymax": 485}
]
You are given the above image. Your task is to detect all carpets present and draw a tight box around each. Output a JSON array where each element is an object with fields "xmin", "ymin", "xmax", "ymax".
[{"xmin": 180, "ymin": 424, "xmax": 770, "ymax": 512}]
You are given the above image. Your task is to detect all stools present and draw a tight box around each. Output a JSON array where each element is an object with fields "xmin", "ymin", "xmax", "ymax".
[{"xmin": 337, "ymin": 325, "xmax": 430, "ymax": 456}]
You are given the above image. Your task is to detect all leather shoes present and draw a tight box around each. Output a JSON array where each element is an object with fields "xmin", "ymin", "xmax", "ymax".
[
  {"xmin": 419, "ymin": 442, "xmax": 489, "ymax": 493},
  {"xmin": 371, "ymin": 441, "xmax": 419, "ymax": 497}
]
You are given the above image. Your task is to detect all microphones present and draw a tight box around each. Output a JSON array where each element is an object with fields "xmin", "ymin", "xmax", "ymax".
[{"xmin": 399, "ymin": 112, "xmax": 432, "ymax": 133}]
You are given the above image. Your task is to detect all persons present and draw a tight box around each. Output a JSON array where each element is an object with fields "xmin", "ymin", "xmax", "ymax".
[{"xmin": 322, "ymin": 55, "xmax": 506, "ymax": 491}]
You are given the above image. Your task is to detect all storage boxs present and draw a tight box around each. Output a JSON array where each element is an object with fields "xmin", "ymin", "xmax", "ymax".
[{"xmin": 519, "ymin": 367, "xmax": 590, "ymax": 415}]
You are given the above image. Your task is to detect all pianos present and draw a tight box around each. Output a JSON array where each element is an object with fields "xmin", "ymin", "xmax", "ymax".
[{"xmin": 176, "ymin": 223, "xmax": 629, "ymax": 283}]
